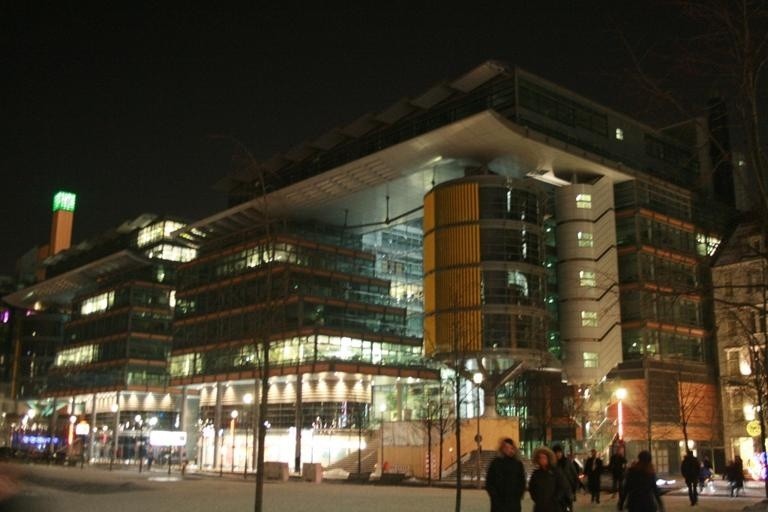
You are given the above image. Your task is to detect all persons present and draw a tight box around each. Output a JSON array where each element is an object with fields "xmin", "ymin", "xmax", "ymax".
[
  {"xmin": 484, "ymin": 437, "xmax": 527, "ymax": 511},
  {"xmin": 527, "ymin": 446, "xmax": 574, "ymax": 512},
  {"xmin": 550, "ymin": 443, "xmax": 665, "ymax": 512},
  {"xmin": 681, "ymin": 451, "xmax": 746, "ymax": 505}
]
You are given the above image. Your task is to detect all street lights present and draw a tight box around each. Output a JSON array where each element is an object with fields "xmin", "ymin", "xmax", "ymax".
[
  {"xmin": 1, "ymin": 392, "xmax": 259, "ymax": 481},
  {"xmin": 470, "ymin": 370, "xmax": 485, "ymax": 492},
  {"xmin": 186, "ymin": 124, "xmax": 281, "ymax": 512},
  {"xmin": 612, "ymin": 385, "xmax": 630, "ymax": 465}
]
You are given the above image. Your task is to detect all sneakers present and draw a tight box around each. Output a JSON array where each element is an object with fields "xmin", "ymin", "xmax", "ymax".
[{"xmin": 591, "ymin": 495, "xmax": 618, "ymax": 504}]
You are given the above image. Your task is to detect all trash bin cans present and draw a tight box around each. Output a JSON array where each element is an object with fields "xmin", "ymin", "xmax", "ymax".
[
  {"xmin": 264, "ymin": 462, "xmax": 289, "ymax": 483},
  {"xmin": 303, "ymin": 463, "xmax": 322, "ymax": 484}
]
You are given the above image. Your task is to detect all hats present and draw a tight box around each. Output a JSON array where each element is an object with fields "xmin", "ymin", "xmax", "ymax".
[{"xmin": 638, "ymin": 450, "xmax": 651, "ymax": 463}]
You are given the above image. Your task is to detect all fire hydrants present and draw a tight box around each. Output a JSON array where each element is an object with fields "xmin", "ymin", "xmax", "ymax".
[{"xmin": 380, "ymin": 460, "xmax": 391, "ymax": 475}]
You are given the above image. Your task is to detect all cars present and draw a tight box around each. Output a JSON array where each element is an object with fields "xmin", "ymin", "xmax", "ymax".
[{"xmin": 574, "ymin": 464, "xmax": 680, "ymax": 499}]
